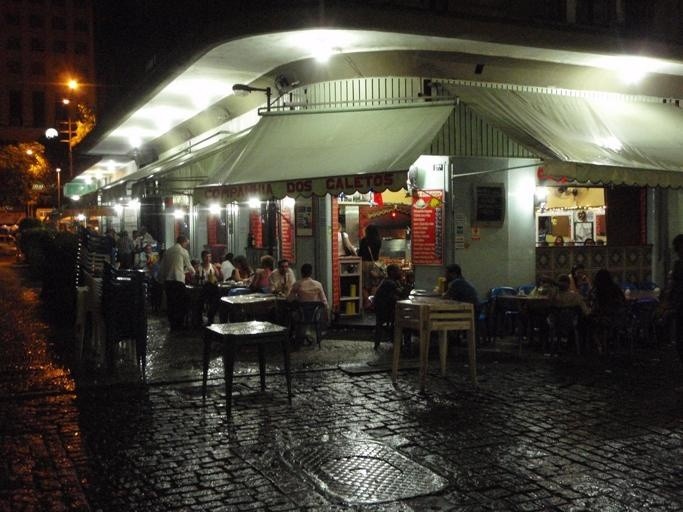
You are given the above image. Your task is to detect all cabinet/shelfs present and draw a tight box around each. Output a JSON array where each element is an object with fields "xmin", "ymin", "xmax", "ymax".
[{"xmin": 338, "ymin": 256, "xmax": 363, "ymax": 317}]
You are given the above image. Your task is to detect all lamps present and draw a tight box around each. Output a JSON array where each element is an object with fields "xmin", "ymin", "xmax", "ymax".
[{"xmin": 232, "ymin": 83, "xmax": 270, "ymax": 113}]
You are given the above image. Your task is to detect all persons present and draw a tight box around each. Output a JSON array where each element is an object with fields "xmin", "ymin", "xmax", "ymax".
[
  {"xmin": 266, "ymin": 259, "xmax": 296, "ymax": 296},
  {"xmin": 660, "ymin": 231, "xmax": 681, "ymax": 395},
  {"xmin": 548, "ymin": 274, "xmax": 592, "ymax": 354},
  {"xmin": 526, "ymin": 275, "xmax": 555, "ymax": 296},
  {"xmin": 158, "ymin": 234, "xmax": 195, "ymax": 330},
  {"xmin": 102, "ymin": 226, "xmax": 158, "ymax": 269},
  {"xmin": 583, "ymin": 268, "xmax": 626, "ymax": 353},
  {"xmin": 282, "ymin": 263, "xmax": 329, "ymax": 345},
  {"xmin": 436, "ymin": 264, "xmax": 482, "ymax": 334},
  {"xmin": 372, "ymin": 264, "xmax": 420, "ymax": 359},
  {"xmin": 356, "ymin": 224, "xmax": 381, "ymax": 311},
  {"xmin": 337, "ymin": 221, "xmax": 357, "ymax": 257},
  {"xmin": 566, "ymin": 263, "xmax": 592, "ymax": 296},
  {"xmin": 247, "ymin": 255, "xmax": 274, "ymax": 291},
  {"xmin": 184, "ymin": 248, "xmax": 253, "ymax": 326},
  {"xmin": 539, "ymin": 237, "xmax": 605, "ymax": 247}
]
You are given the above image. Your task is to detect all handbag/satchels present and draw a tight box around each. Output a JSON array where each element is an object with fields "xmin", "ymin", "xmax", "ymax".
[
  {"xmin": 369, "ymin": 266, "xmax": 385, "ymax": 287},
  {"xmin": 344, "ymin": 245, "xmax": 352, "ymax": 256}
]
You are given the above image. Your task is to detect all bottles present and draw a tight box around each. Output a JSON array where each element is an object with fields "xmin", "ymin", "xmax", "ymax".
[{"xmin": 194, "ymin": 270, "xmax": 206, "ymax": 287}]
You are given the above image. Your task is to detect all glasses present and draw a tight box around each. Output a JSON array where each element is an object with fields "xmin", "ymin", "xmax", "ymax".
[{"xmin": 281, "ymin": 265, "xmax": 288, "ymax": 268}]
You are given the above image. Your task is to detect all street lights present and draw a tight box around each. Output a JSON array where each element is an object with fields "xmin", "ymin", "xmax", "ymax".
[
  {"xmin": 56, "ymin": 168, "xmax": 61, "ymax": 212},
  {"xmin": 63, "ymin": 99, "xmax": 75, "ymax": 175}
]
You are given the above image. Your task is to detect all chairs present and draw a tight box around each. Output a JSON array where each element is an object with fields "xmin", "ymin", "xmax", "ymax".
[
  {"xmin": 367, "ymin": 295, "xmax": 395, "ymax": 350},
  {"xmin": 283, "ymin": 300, "xmax": 324, "ymax": 351},
  {"xmin": 76, "ymin": 223, "xmax": 148, "ymax": 378},
  {"xmin": 477, "ymin": 281, "xmax": 657, "ymax": 366}
]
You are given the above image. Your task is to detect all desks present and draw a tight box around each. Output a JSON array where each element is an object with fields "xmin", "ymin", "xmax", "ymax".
[
  {"xmin": 393, "ymin": 298, "xmax": 479, "ymax": 394},
  {"xmin": 200, "ymin": 291, "xmax": 293, "ymax": 421}
]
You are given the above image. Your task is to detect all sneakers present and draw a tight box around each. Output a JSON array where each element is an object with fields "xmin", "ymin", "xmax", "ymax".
[{"xmin": 364, "ymin": 300, "xmax": 372, "ymax": 309}]
